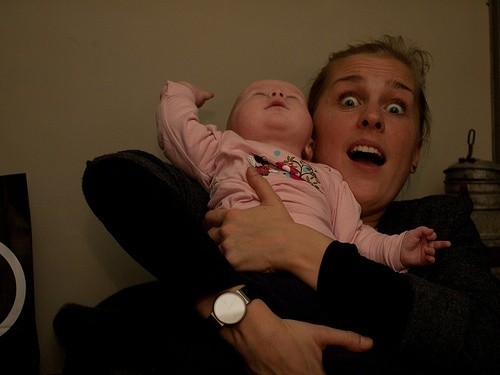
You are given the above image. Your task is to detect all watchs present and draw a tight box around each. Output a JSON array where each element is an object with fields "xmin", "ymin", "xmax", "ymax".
[{"xmin": 205, "ymin": 284, "xmax": 260, "ymax": 334}]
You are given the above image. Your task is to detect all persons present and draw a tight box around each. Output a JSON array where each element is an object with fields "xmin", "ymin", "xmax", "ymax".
[
  {"xmin": 157, "ymin": 75, "xmax": 458, "ymax": 319},
  {"xmin": 80, "ymin": 35, "xmax": 500, "ymax": 374}
]
